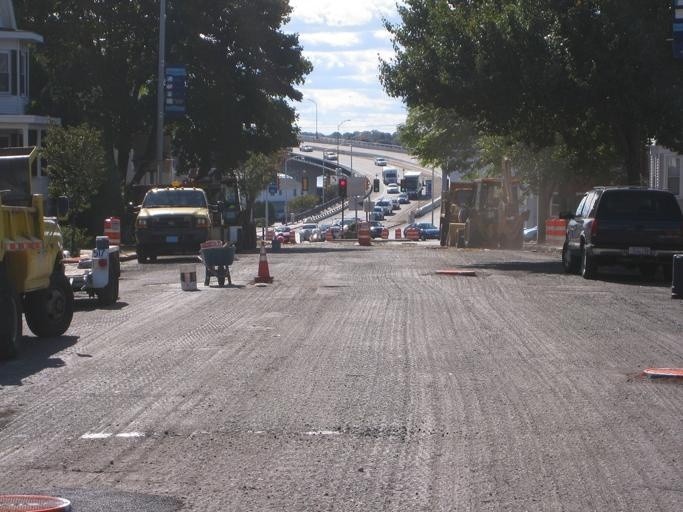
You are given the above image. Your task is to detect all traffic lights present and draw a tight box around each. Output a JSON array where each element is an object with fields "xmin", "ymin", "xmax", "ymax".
[{"xmin": 339, "ymin": 179, "xmax": 347, "ymax": 197}]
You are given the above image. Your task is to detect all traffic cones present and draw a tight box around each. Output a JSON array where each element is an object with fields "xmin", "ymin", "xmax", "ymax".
[{"xmin": 255, "ymin": 239, "xmax": 274, "ymax": 284}]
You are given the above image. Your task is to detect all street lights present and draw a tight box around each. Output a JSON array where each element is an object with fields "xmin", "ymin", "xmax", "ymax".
[
  {"xmin": 284, "ymin": 153, "xmax": 306, "ymax": 226},
  {"xmin": 375, "ymin": 157, "xmax": 387, "ymax": 165},
  {"xmin": 336, "ymin": 119, "xmax": 352, "ymax": 168},
  {"xmin": 382, "ymin": 166, "xmax": 398, "ymax": 184},
  {"xmin": 306, "ymin": 97, "xmax": 319, "ymax": 139}
]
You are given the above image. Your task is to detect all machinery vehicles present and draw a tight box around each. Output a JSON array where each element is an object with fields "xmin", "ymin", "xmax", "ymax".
[{"xmin": 440, "ymin": 156, "xmax": 530, "ymax": 250}]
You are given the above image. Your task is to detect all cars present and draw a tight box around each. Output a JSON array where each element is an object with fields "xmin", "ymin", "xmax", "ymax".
[
  {"xmin": 298, "ymin": 143, "xmax": 313, "ymax": 151},
  {"xmin": 403, "ymin": 222, "xmax": 440, "ymax": 241},
  {"xmin": 323, "ymin": 151, "xmax": 338, "ymax": 160},
  {"xmin": 256, "ymin": 195, "xmax": 409, "ymax": 253},
  {"xmin": 388, "ymin": 183, "xmax": 401, "ymax": 193}
]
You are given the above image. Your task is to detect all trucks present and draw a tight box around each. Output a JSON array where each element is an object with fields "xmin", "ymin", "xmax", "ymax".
[
  {"xmin": 402, "ymin": 171, "xmax": 424, "ymax": 199},
  {"xmin": 0, "ymin": 147, "xmax": 75, "ymax": 362}
]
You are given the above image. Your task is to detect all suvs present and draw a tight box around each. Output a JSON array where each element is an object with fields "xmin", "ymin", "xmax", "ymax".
[
  {"xmin": 558, "ymin": 186, "xmax": 683, "ymax": 279},
  {"xmin": 128, "ymin": 187, "xmax": 223, "ymax": 264}
]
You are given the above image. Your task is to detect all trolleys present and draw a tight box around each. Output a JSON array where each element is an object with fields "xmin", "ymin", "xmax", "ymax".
[{"xmin": 195, "ymin": 244, "xmax": 237, "ymax": 287}]
